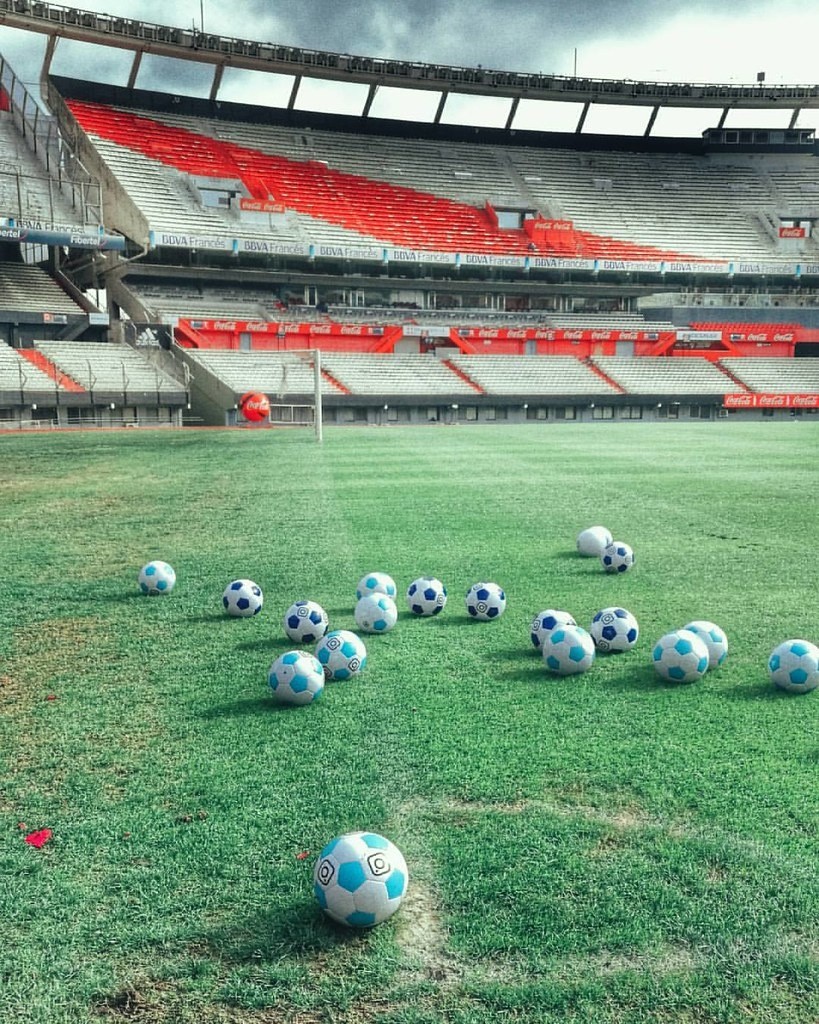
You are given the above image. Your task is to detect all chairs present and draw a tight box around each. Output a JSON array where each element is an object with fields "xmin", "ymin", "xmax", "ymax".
[{"xmin": 0, "ymin": 82, "xmax": 819, "ymax": 398}]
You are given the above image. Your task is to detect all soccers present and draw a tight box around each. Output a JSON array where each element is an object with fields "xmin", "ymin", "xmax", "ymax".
[
  {"xmin": 136, "ymin": 521, "xmax": 819, "ymax": 706},
  {"xmin": 313, "ymin": 831, "xmax": 408, "ymax": 929},
  {"xmin": 239, "ymin": 390, "xmax": 271, "ymax": 422}
]
